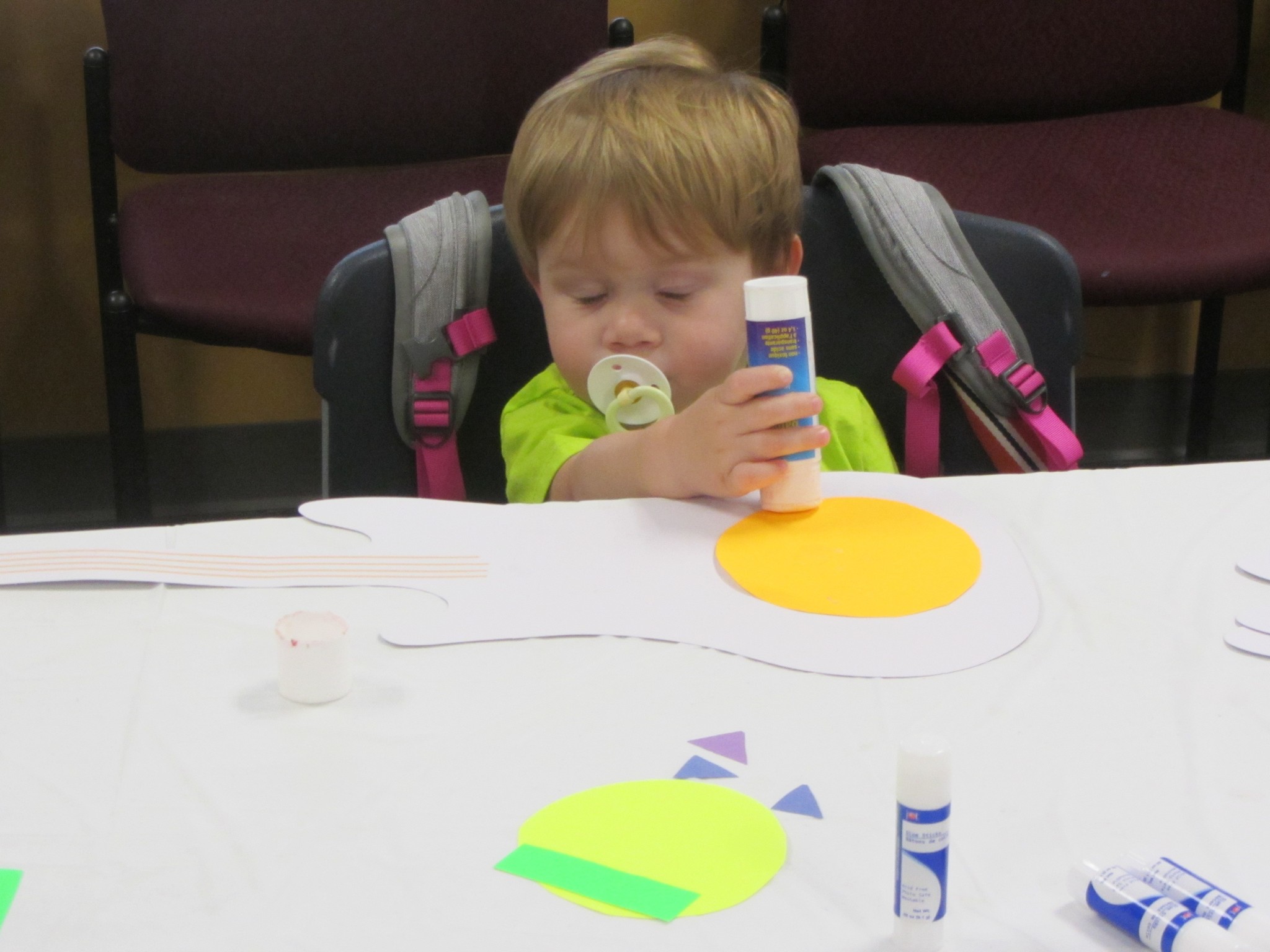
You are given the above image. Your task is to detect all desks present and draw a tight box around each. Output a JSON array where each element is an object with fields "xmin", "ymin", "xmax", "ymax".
[{"xmin": 0, "ymin": 459, "xmax": 1269, "ymax": 951}]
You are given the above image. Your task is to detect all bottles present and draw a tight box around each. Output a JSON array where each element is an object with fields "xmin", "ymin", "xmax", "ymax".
[
  {"xmin": 742, "ymin": 274, "xmax": 824, "ymax": 513},
  {"xmin": 891, "ymin": 777, "xmax": 951, "ymax": 947},
  {"xmin": 1069, "ymin": 847, "xmax": 1261, "ymax": 951}
]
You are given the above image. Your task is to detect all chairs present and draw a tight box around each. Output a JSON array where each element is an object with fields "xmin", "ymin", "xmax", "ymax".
[{"xmin": 81, "ymin": 0, "xmax": 1270, "ymax": 517}]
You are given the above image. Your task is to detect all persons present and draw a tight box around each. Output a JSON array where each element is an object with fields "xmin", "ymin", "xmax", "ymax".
[{"xmin": 501, "ymin": 32, "xmax": 900, "ymax": 500}]
